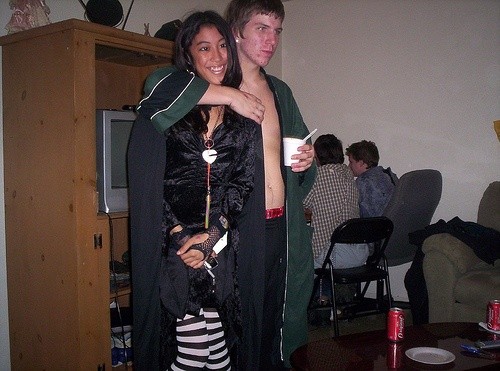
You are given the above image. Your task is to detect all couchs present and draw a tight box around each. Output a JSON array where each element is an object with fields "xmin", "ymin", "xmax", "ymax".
[{"xmin": 403, "ymin": 180, "xmax": 500, "ymax": 325}]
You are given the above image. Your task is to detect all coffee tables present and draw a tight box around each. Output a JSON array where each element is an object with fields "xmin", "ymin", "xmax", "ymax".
[{"xmin": 289, "ymin": 321, "xmax": 500, "ymax": 371}]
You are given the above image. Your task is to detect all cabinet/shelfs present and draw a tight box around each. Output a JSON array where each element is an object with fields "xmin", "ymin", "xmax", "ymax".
[{"xmin": 0, "ymin": 18, "xmax": 174, "ymax": 371}]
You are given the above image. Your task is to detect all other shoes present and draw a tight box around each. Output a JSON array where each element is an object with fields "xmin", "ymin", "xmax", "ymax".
[{"xmin": 307, "ymin": 315, "xmax": 332, "ymax": 328}]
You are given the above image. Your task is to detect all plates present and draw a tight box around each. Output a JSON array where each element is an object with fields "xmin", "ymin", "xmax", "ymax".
[{"xmin": 405, "ymin": 347, "xmax": 456, "ymax": 365}]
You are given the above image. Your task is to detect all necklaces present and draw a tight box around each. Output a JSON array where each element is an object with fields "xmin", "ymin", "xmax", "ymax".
[{"xmin": 201, "ymin": 105, "xmax": 224, "ymax": 229}]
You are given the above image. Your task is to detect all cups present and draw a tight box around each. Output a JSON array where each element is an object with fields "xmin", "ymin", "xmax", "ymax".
[{"xmin": 283, "ymin": 137, "xmax": 306, "ymax": 167}]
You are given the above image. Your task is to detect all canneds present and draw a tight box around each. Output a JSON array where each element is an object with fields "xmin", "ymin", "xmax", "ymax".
[
  {"xmin": 487, "ymin": 300, "xmax": 500, "ymax": 331},
  {"xmin": 387, "ymin": 343, "xmax": 402, "ymax": 370},
  {"xmin": 387, "ymin": 308, "xmax": 405, "ymax": 342}
]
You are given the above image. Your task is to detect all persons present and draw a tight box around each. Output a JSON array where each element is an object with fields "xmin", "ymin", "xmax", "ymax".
[
  {"xmin": 302, "ymin": 133, "xmax": 370, "ymax": 329},
  {"xmin": 334, "ymin": 140, "xmax": 399, "ymax": 303},
  {"xmin": 126, "ymin": 9, "xmax": 266, "ymax": 371},
  {"xmin": 137, "ymin": 0, "xmax": 319, "ymax": 371}
]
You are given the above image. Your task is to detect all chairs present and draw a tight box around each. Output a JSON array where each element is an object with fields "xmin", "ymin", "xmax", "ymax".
[
  {"xmin": 307, "ymin": 216, "xmax": 394, "ymax": 340},
  {"xmin": 374, "ymin": 169, "xmax": 444, "ymax": 311}
]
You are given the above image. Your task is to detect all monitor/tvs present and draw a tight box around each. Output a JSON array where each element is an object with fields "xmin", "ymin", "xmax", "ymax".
[{"xmin": 95, "ymin": 109, "xmax": 129, "ymax": 214}]
